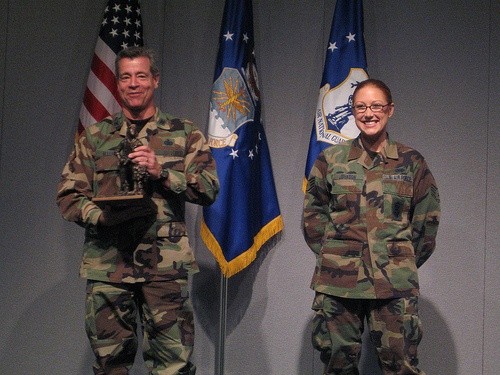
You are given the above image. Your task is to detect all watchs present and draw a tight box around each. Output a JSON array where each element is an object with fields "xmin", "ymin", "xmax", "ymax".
[{"xmin": 158, "ymin": 168, "xmax": 168, "ymax": 182}]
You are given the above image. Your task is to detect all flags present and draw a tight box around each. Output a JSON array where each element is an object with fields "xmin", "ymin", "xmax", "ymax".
[
  {"xmin": 75, "ymin": 0, "xmax": 142, "ymax": 146},
  {"xmin": 199, "ymin": 0, "xmax": 284, "ymax": 277},
  {"xmin": 302, "ymin": 0, "xmax": 367, "ymax": 194}
]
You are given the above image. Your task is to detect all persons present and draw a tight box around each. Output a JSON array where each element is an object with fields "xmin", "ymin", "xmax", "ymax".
[
  {"xmin": 301, "ymin": 79, "xmax": 440, "ymax": 375},
  {"xmin": 115, "ymin": 124, "xmax": 147, "ymax": 194},
  {"xmin": 56, "ymin": 48, "xmax": 219, "ymax": 375}
]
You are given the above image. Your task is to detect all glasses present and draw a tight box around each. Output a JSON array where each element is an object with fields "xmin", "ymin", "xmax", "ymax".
[{"xmin": 352, "ymin": 103, "xmax": 391, "ymax": 113}]
